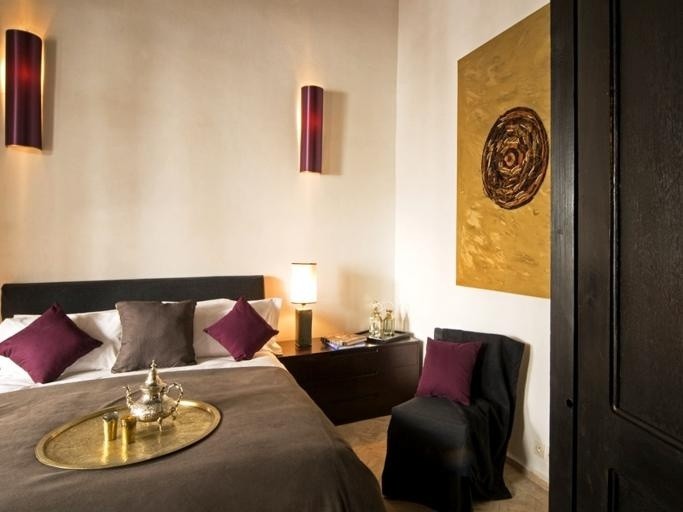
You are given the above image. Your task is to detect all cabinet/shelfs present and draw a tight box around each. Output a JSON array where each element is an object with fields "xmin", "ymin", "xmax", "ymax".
[{"xmin": 277, "ymin": 333, "xmax": 422, "ymax": 427}]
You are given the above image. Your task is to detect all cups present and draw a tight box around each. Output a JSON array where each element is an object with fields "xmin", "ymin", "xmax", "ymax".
[
  {"xmin": 121, "ymin": 443, "xmax": 136, "ymax": 463},
  {"xmin": 101, "ymin": 441, "xmax": 119, "ymax": 466},
  {"xmin": 120, "ymin": 417, "xmax": 136, "ymax": 444},
  {"xmin": 101, "ymin": 412, "xmax": 118, "ymax": 441}
]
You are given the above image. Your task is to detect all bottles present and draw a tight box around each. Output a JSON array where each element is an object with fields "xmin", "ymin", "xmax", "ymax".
[
  {"xmin": 368, "ymin": 300, "xmax": 382, "ymax": 338},
  {"xmin": 382, "ymin": 302, "xmax": 396, "ymax": 335}
]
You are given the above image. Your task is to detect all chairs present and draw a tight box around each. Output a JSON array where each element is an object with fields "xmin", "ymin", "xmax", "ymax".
[{"xmin": 378, "ymin": 326, "xmax": 525, "ymax": 512}]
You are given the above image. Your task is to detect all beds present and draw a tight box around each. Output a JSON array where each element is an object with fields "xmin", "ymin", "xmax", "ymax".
[{"xmin": 0, "ymin": 273, "xmax": 386, "ymax": 510}]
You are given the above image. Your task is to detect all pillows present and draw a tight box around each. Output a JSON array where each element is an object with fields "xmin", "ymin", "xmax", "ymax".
[
  {"xmin": 0, "ymin": 291, "xmax": 281, "ymax": 386},
  {"xmin": 414, "ymin": 336, "xmax": 484, "ymax": 406}
]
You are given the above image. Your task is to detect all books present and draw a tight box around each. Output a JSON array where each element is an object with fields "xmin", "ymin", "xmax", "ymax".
[{"xmin": 320, "ymin": 331, "xmax": 367, "ymax": 351}]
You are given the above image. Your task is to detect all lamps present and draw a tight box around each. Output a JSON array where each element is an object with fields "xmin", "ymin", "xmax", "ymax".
[
  {"xmin": 290, "ymin": 262, "xmax": 318, "ymax": 349},
  {"xmin": 298, "ymin": 85, "xmax": 323, "ymax": 175},
  {"xmin": 3, "ymin": 29, "xmax": 42, "ymax": 153}
]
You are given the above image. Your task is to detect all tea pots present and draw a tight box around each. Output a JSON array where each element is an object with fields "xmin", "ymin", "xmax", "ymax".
[{"xmin": 122, "ymin": 357, "xmax": 183, "ymax": 431}]
